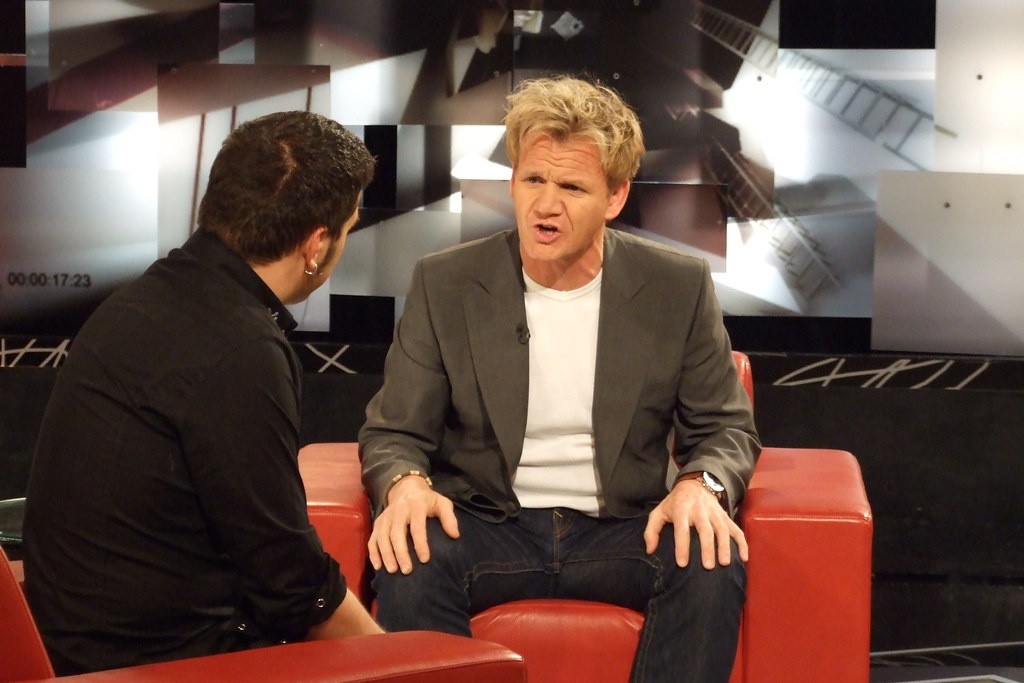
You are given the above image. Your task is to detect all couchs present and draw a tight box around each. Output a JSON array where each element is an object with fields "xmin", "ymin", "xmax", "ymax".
[
  {"xmin": 0, "ymin": 545, "xmax": 527, "ymax": 683},
  {"xmin": 299, "ymin": 351, "xmax": 872, "ymax": 683}
]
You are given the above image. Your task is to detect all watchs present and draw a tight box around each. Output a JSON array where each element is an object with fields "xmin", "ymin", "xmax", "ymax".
[{"xmin": 672, "ymin": 470, "xmax": 726, "ymax": 502}]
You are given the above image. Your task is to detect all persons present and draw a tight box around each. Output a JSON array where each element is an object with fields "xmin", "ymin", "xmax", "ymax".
[
  {"xmin": 18, "ymin": 110, "xmax": 388, "ymax": 676},
  {"xmin": 358, "ymin": 72, "xmax": 762, "ymax": 683}
]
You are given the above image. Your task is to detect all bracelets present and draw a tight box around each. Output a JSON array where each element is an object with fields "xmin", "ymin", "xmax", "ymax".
[{"xmin": 385, "ymin": 470, "xmax": 434, "ymax": 501}]
